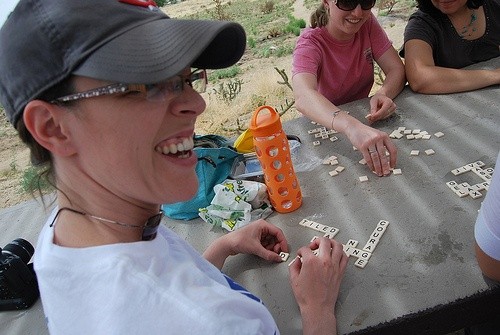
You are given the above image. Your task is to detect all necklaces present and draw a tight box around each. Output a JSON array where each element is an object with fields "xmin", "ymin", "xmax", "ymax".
[{"xmin": 459, "ymin": 11, "xmax": 477, "ymax": 39}]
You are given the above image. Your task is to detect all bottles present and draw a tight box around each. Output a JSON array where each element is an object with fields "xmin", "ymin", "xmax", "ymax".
[{"xmin": 248, "ymin": 106, "xmax": 302, "ymax": 214}]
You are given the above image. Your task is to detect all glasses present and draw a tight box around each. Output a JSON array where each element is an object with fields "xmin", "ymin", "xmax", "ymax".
[
  {"xmin": 333, "ymin": 0, "xmax": 376, "ymax": 12},
  {"xmin": 50, "ymin": 68, "xmax": 208, "ymax": 105},
  {"xmin": 49, "ymin": 207, "xmax": 166, "ymax": 241}
]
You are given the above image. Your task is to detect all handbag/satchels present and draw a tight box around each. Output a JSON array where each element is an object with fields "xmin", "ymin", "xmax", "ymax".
[{"xmin": 162, "ymin": 134, "xmax": 243, "ymax": 221}]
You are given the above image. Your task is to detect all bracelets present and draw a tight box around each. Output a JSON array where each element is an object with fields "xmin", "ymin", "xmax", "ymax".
[{"xmin": 331, "ymin": 109, "xmax": 350, "ymax": 130}]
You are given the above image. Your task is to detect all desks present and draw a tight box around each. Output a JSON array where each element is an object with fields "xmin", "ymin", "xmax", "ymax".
[{"xmin": 1, "ymin": 52, "xmax": 500, "ymax": 334}]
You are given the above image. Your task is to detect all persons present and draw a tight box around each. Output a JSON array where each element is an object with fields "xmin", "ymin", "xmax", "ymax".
[
  {"xmin": 293, "ymin": 0, "xmax": 407, "ymax": 178},
  {"xmin": 473, "ymin": 147, "xmax": 500, "ymax": 282},
  {"xmin": 399, "ymin": 0, "xmax": 500, "ymax": 96},
  {"xmin": 0, "ymin": 0, "xmax": 348, "ymax": 335}
]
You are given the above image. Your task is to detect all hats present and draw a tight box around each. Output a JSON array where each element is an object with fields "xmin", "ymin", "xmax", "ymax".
[{"xmin": 0, "ymin": 0, "xmax": 247, "ymax": 128}]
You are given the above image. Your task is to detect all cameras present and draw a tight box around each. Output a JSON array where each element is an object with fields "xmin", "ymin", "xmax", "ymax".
[{"xmin": 0, "ymin": 237, "xmax": 40, "ymax": 311}]
